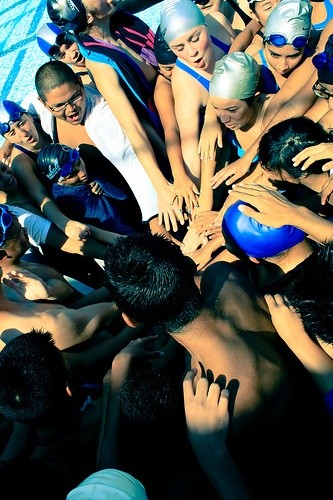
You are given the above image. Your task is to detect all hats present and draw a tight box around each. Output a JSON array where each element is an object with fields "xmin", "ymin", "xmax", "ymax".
[
  {"xmin": 0, "ymin": 100, "xmax": 24, "ymax": 127},
  {"xmin": 263, "ymin": 0, "xmax": 314, "ymax": 44},
  {"xmin": 154, "ymin": 25, "xmax": 177, "ymax": 65},
  {"xmin": 37, "ymin": 143, "xmax": 72, "ymax": 183},
  {"xmin": 159, "ymin": 0, "xmax": 208, "ymax": 44},
  {"xmin": 47, "ymin": 0, "xmax": 87, "ymax": 30},
  {"xmin": 318, "ymin": 33, "xmax": 333, "ymax": 85},
  {"xmin": 36, "ymin": 22, "xmax": 64, "ymax": 58},
  {"xmin": 209, "ymin": 51, "xmax": 261, "ymax": 100},
  {"xmin": 221, "ymin": 200, "xmax": 306, "ymax": 259}
]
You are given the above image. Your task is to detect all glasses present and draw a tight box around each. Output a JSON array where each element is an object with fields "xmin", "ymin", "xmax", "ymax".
[
  {"xmin": 49, "ymin": 30, "xmax": 69, "ymax": 59},
  {"xmin": 0, "ymin": 204, "xmax": 13, "ymax": 248},
  {"xmin": 42, "ymin": 79, "xmax": 83, "ymax": 112},
  {"xmin": 312, "ymin": 79, "xmax": 333, "ymax": 100},
  {"xmin": 153, "ymin": 41, "xmax": 172, "ymax": 54},
  {"xmin": 312, "ymin": 53, "xmax": 333, "ymax": 73},
  {"xmin": 58, "ymin": 148, "xmax": 80, "ymax": 182},
  {"xmin": 263, "ymin": 34, "xmax": 308, "ymax": 50},
  {"xmin": 248, "ymin": 0, "xmax": 262, "ymax": 4},
  {"xmin": 0, "ymin": 111, "xmax": 28, "ymax": 137}
]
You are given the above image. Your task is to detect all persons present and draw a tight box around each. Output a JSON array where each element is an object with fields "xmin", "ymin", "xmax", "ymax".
[
  {"xmin": 47, "ymin": 0, "xmax": 189, "ymax": 232},
  {"xmin": 103, "ymin": 229, "xmax": 333, "ymax": 500},
  {"xmin": 36, "ymin": 60, "xmax": 187, "ymax": 248},
  {"xmin": 0, "ymin": 0, "xmax": 333, "ymax": 500},
  {"xmin": 160, "ymin": 0, "xmax": 237, "ymax": 192}
]
